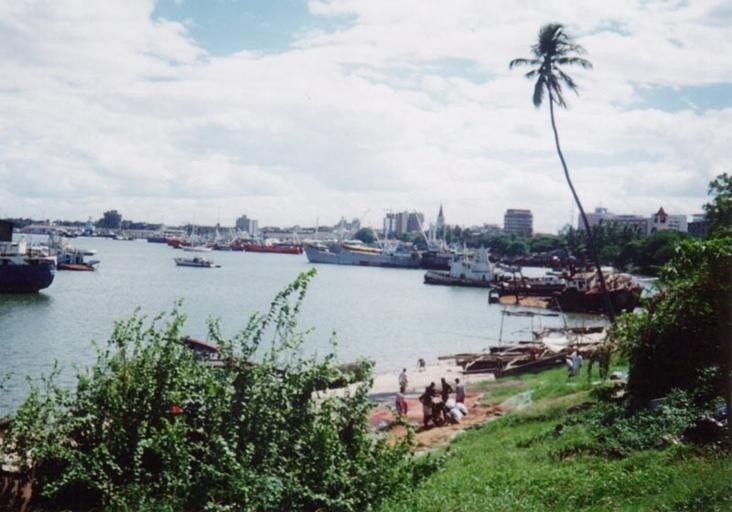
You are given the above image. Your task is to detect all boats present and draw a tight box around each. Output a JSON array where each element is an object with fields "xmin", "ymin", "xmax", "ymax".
[
  {"xmin": 0, "ymin": 219, "xmax": 137, "ymax": 293},
  {"xmin": 438, "ymin": 316, "xmax": 621, "ymax": 378}
]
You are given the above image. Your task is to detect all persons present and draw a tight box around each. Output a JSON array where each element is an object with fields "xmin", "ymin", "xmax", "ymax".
[
  {"xmin": 416, "ymin": 359, "xmax": 425, "ymax": 370},
  {"xmin": 398, "ymin": 368, "xmax": 409, "ymax": 386},
  {"xmin": 417, "ymin": 378, "xmax": 466, "ymax": 429},
  {"xmin": 396, "ymin": 387, "xmax": 408, "ymax": 419},
  {"xmin": 564, "ymin": 357, "xmax": 573, "ymax": 376},
  {"xmin": 574, "ymin": 351, "xmax": 584, "ymax": 377},
  {"xmin": 567, "ymin": 345, "xmax": 578, "ymax": 362}
]
